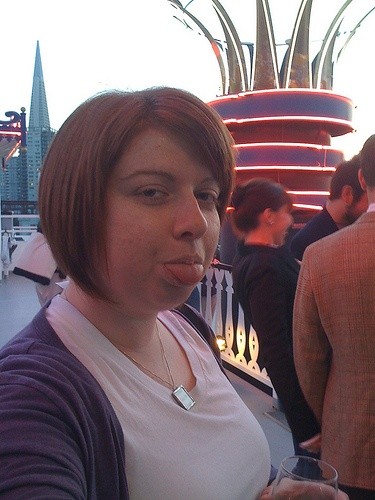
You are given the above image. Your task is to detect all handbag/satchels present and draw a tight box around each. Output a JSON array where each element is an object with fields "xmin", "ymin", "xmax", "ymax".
[{"xmin": 11, "ymin": 232, "xmax": 58, "ymax": 285}]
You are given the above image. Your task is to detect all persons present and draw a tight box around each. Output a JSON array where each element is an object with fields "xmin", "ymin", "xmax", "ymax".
[
  {"xmin": 291, "ymin": 158, "xmax": 368, "ymax": 262},
  {"xmin": 35, "ymin": 219, "xmax": 67, "ymax": 307},
  {"xmin": 230, "ymin": 178, "xmax": 324, "ymax": 478},
  {"xmin": 292, "ymin": 134, "xmax": 375, "ymax": 500},
  {"xmin": 0, "ymin": 86, "xmax": 348, "ymax": 500},
  {"xmin": 185, "ymin": 210, "xmax": 252, "ymax": 362}
]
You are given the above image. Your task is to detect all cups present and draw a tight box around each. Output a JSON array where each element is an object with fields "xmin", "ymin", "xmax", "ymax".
[{"xmin": 270, "ymin": 455, "xmax": 339, "ymax": 500}]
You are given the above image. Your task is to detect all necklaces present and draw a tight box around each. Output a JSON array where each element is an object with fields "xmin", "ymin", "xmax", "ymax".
[{"xmin": 63, "ymin": 289, "xmax": 196, "ymax": 412}]
